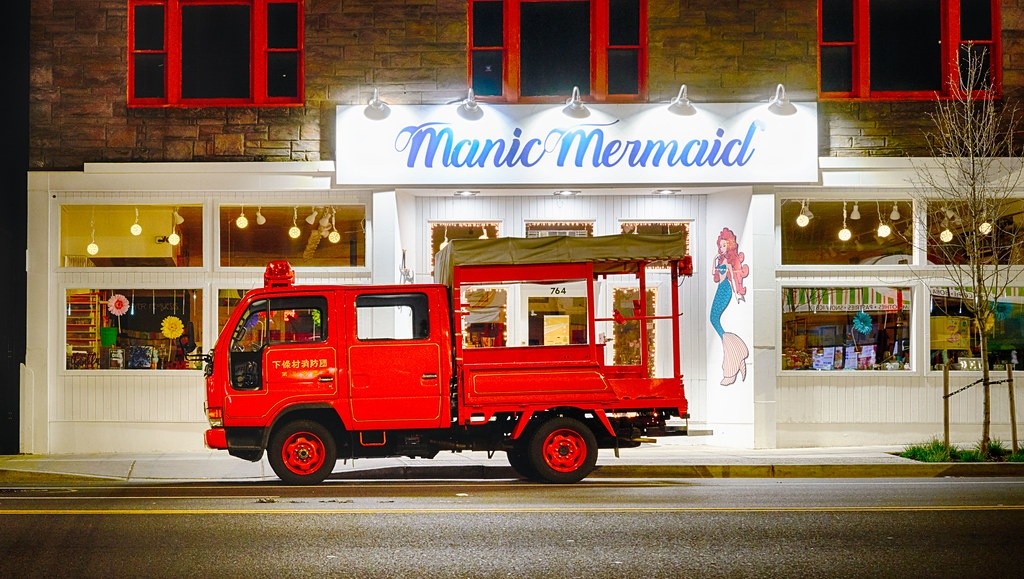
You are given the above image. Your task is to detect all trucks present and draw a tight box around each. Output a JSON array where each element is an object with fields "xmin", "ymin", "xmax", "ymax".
[{"xmin": 178, "ymin": 230, "xmax": 694, "ymax": 486}]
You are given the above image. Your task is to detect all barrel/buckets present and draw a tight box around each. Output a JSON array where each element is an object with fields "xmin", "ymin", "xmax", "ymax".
[{"xmin": 101, "ymin": 327, "xmax": 117, "ymax": 347}]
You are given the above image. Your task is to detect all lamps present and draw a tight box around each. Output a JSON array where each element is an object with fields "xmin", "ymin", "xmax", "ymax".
[
  {"xmin": 236, "ymin": 207, "xmax": 248, "ymax": 229},
  {"xmin": 796, "ymin": 199, "xmax": 963, "ymax": 258},
  {"xmin": 328, "ymin": 207, "xmax": 341, "ymax": 244},
  {"xmin": 319, "ymin": 207, "xmax": 331, "ymax": 226},
  {"xmin": 979, "ymin": 200, "xmax": 992, "ymax": 235},
  {"xmin": 288, "ymin": 207, "xmax": 301, "ymax": 239},
  {"xmin": 479, "ymin": 226, "xmax": 489, "ymax": 239},
  {"xmin": 164, "ymin": 205, "xmax": 185, "ymax": 245},
  {"xmin": 306, "ymin": 207, "xmax": 318, "ymax": 225},
  {"xmin": 667, "ymin": 84, "xmax": 698, "ymax": 118},
  {"xmin": 562, "ymin": 86, "xmax": 591, "ymax": 119},
  {"xmin": 439, "ymin": 224, "xmax": 449, "ymax": 251},
  {"xmin": 456, "ymin": 89, "xmax": 484, "ymax": 120},
  {"xmin": 363, "ymin": 86, "xmax": 391, "ymax": 120},
  {"xmin": 256, "ymin": 206, "xmax": 266, "ymax": 225},
  {"xmin": 87, "ymin": 206, "xmax": 99, "ymax": 256},
  {"xmin": 130, "ymin": 206, "xmax": 143, "ymax": 235},
  {"xmin": 769, "ymin": 83, "xmax": 799, "ymax": 118}
]
